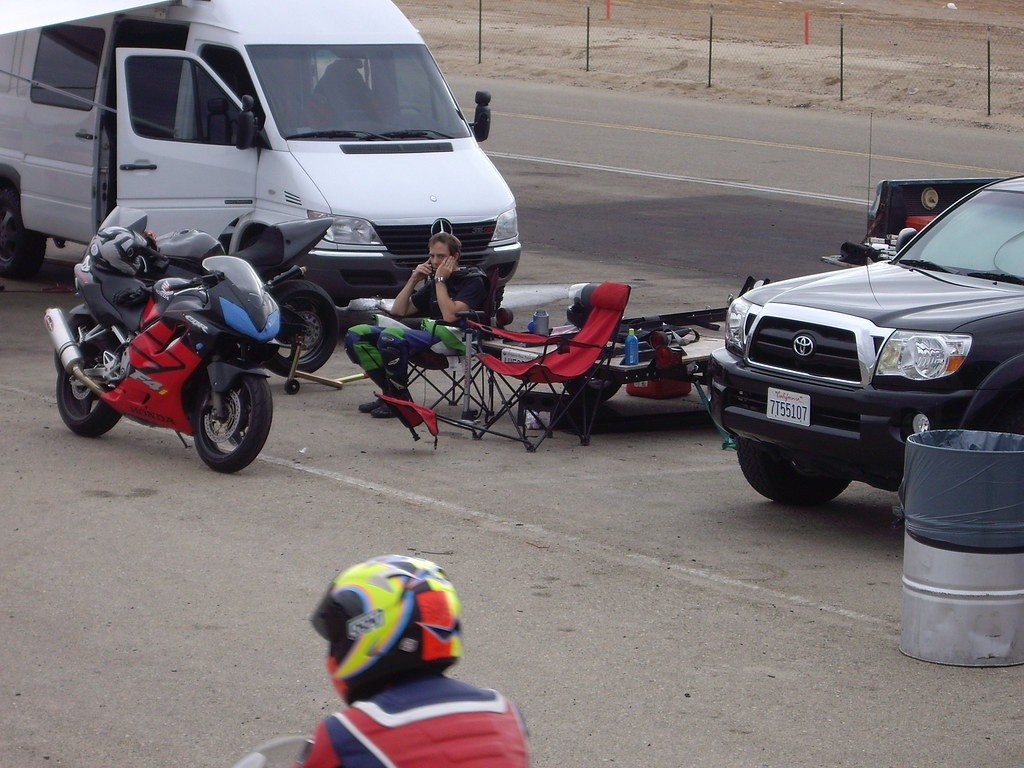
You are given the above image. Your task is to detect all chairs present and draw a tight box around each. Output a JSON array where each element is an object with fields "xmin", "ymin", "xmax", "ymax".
[
  {"xmin": 372, "ymin": 281, "xmax": 632, "ymax": 452},
  {"xmin": 304, "ymin": 60, "xmax": 382, "ymax": 128},
  {"xmin": 374, "ymin": 263, "xmax": 503, "ymax": 411}
]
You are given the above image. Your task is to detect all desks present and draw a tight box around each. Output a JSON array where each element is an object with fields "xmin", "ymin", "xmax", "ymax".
[{"xmin": 474, "ymin": 325, "xmax": 727, "ymax": 444}]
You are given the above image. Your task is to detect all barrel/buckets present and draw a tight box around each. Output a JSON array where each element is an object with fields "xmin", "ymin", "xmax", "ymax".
[{"xmin": 898, "ymin": 429, "xmax": 1024, "ymax": 668}]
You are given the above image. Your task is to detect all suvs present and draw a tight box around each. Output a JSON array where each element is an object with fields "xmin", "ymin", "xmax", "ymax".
[{"xmin": 705, "ymin": 172, "xmax": 1023, "ymax": 510}]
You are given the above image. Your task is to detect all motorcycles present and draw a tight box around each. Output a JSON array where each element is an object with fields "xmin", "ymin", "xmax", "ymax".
[{"xmin": 46, "ymin": 208, "xmax": 342, "ymax": 475}]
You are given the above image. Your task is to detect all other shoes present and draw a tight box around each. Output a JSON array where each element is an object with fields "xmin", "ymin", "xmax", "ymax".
[
  {"xmin": 359, "ymin": 390, "xmax": 404, "ymax": 413},
  {"xmin": 371, "ymin": 389, "xmax": 415, "ymax": 417}
]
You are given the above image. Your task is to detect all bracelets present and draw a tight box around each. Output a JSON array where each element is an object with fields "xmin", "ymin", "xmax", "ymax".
[{"xmin": 434, "ymin": 277, "xmax": 446, "ymax": 283}]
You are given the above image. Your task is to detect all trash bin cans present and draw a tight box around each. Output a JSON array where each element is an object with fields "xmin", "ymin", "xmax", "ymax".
[{"xmin": 898, "ymin": 429, "xmax": 1024, "ymax": 668}]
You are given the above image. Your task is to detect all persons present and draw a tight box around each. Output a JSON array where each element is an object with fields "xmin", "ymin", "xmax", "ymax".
[
  {"xmin": 291, "ymin": 554, "xmax": 532, "ymax": 768},
  {"xmin": 344, "ymin": 232, "xmax": 489, "ymax": 418}
]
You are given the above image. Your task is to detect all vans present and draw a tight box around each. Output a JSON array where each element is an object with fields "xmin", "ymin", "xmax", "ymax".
[{"xmin": 0, "ymin": 1, "xmax": 521, "ymax": 311}]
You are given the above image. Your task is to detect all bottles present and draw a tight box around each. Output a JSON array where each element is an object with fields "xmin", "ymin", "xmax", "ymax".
[
  {"xmin": 625, "ymin": 329, "xmax": 638, "ymax": 365},
  {"xmin": 533, "ymin": 307, "xmax": 548, "ymax": 336}
]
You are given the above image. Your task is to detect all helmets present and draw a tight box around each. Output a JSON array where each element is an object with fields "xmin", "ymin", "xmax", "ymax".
[
  {"xmin": 88, "ymin": 225, "xmax": 149, "ymax": 275},
  {"xmin": 310, "ymin": 554, "xmax": 462, "ymax": 700}
]
